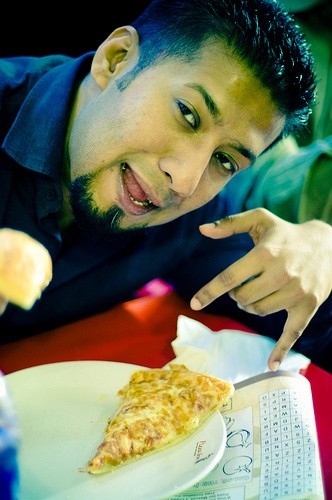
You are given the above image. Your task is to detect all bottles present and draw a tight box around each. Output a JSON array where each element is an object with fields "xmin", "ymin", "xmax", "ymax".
[{"xmin": 0, "ymin": 370, "xmax": 21, "ymax": 500}]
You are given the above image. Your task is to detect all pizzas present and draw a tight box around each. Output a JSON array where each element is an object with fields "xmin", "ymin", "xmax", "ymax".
[{"xmin": 77, "ymin": 363, "xmax": 234, "ymax": 475}]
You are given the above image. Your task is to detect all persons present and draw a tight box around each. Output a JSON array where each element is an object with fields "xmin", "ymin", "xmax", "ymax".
[{"xmin": 0, "ymin": 0, "xmax": 332, "ymax": 373}]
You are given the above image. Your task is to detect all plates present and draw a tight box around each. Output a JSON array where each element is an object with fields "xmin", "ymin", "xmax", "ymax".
[{"xmin": 0, "ymin": 360, "xmax": 227, "ymax": 500}]
